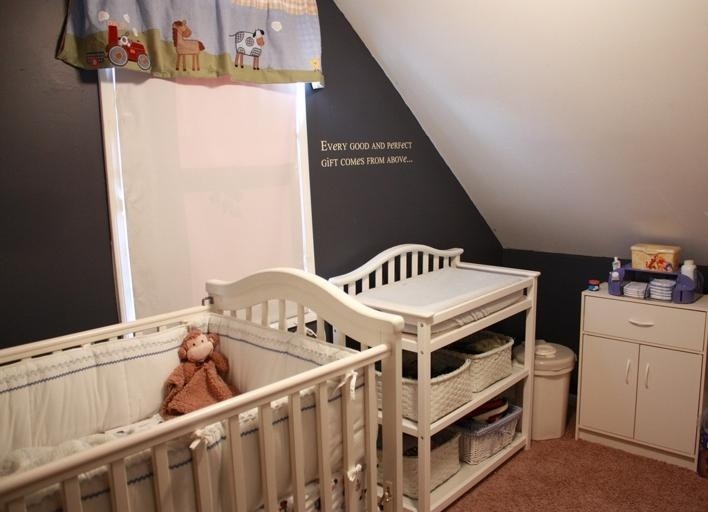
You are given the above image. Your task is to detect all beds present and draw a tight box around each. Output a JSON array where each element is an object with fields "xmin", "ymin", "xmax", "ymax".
[{"xmin": 0, "ymin": 268, "xmax": 404, "ymax": 512}]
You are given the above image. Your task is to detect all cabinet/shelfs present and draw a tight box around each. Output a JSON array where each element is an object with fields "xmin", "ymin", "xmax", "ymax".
[
  {"xmin": 575, "ymin": 280, "xmax": 708, "ymax": 473},
  {"xmin": 324, "ymin": 243, "xmax": 536, "ymax": 511}
]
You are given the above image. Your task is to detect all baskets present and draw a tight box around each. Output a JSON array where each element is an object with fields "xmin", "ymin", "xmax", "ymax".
[
  {"xmin": 448, "ymin": 330, "xmax": 514, "ymax": 392},
  {"xmin": 377, "ymin": 428, "xmax": 461, "ymax": 500},
  {"xmin": 375, "ymin": 351, "xmax": 472, "ymax": 424}
]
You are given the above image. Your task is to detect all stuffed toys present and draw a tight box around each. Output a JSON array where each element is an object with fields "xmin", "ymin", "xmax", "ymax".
[{"xmin": 163, "ymin": 327, "xmax": 233, "ymax": 413}]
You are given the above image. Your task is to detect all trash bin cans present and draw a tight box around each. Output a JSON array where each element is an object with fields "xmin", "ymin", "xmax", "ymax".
[{"xmin": 512, "ymin": 339, "xmax": 578, "ymax": 441}]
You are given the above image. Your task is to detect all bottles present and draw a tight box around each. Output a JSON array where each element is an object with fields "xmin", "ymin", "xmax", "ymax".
[{"xmin": 681, "ymin": 259, "xmax": 698, "ymax": 284}]
